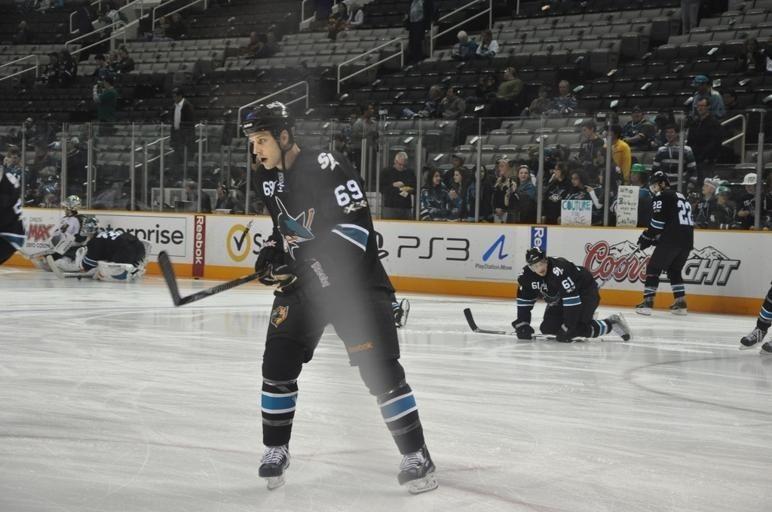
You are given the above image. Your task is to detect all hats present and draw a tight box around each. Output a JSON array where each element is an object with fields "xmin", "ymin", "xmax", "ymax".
[
  {"xmin": 739, "ymin": 172, "xmax": 757, "ymax": 186},
  {"xmin": 690, "ymin": 74, "xmax": 710, "ymax": 87},
  {"xmin": 713, "ymin": 184, "xmax": 732, "ymax": 194},
  {"xmin": 628, "ymin": 162, "xmax": 647, "ymax": 173}
]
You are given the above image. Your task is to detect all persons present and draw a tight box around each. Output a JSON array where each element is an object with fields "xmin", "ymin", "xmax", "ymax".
[
  {"xmin": 1, "ymin": 1, "xmax": 487, "ymax": 215},
  {"xmin": 741, "ymin": 283, "xmax": 772, "ymax": 353},
  {"xmin": 250, "ymin": 28, "xmax": 772, "ymax": 230},
  {"xmin": 381, "ymin": 267, "xmax": 410, "ymax": 327},
  {"xmin": 511, "ymin": 246, "xmax": 631, "ymax": 343},
  {"xmin": 239, "ymin": 102, "xmax": 430, "ymax": 485},
  {"xmin": 635, "ymin": 171, "xmax": 695, "ymax": 317},
  {"xmin": 1, "ymin": 164, "xmax": 147, "ymax": 282}
]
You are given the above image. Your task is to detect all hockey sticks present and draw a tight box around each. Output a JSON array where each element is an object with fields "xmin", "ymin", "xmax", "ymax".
[
  {"xmin": 464, "ymin": 308, "xmax": 586, "ymax": 341},
  {"xmin": 159, "ymin": 252, "xmax": 270, "ymax": 307},
  {"xmin": 47, "ymin": 255, "xmax": 92, "ymax": 278}
]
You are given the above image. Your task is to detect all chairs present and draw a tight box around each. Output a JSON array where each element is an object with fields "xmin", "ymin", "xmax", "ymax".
[{"xmin": 2, "ymin": 1, "xmax": 772, "ymax": 230}]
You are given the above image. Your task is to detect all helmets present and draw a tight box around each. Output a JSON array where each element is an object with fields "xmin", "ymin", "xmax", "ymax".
[
  {"xmin": 60, "ymin": 194, "xmax": 83, "ymax": 212},
  {"xmin": 647, "ymin": 170, "xmax": 671, "ymax": 189},
  {"xmin": 239, "ymin": 100, "xmax": 296, "ymax": 135},
  {"xmin": 525, "ymin": 247, "xmax": 545, "ymax": 266},
  {"xmin": 80, "ymin": 216, "xmax": 99, "ymax": 235}
]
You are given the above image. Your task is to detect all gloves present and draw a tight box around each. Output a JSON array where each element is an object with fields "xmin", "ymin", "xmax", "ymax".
[
  {"xmin": 273, "ymin": 246, "xmax": 343, "ymax": 310},
  {"xmin": 253, "ymin": 233, "xmax": 284, "ymax": 286},
  {"xmin": 555, "ymin": 321, "xmax": 576, "ymax": 343},
  {"xmin": 636, "ymin": 230, "xmax": 658, "ymax": 250},
  {"xmin": 511, "ymin": 317, "xmax": 535, "ymax": 340}
]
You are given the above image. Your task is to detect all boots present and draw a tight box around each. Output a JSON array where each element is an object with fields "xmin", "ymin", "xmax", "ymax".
[
  {"xmin": 740, "ymin": 326, "xmax": 767, "ymax": 347},
  {"xmin": 392, "ymin": 297, "xmax": 404, "ymax": 327},
  {"xmin": 669, "ymin": 298, "xmax": 687, "ymax": 311},
  {"xmin": 610, "ymin": 311, "xmax": 631, "ymax": 341},
  {"xmin": 635, "ymin": 297, "xmax": 654, "ymax": 309},
  {"xmin": 762, "ymin": 340, "xmax": 772, "ymax": 353},
  {"xmin": 397, "ymin": 443, "xmax": 437, "ymax": 487},
  {"xmin": 257, "ymin": 442, "xmax": 292, "ymax": 478}
]
